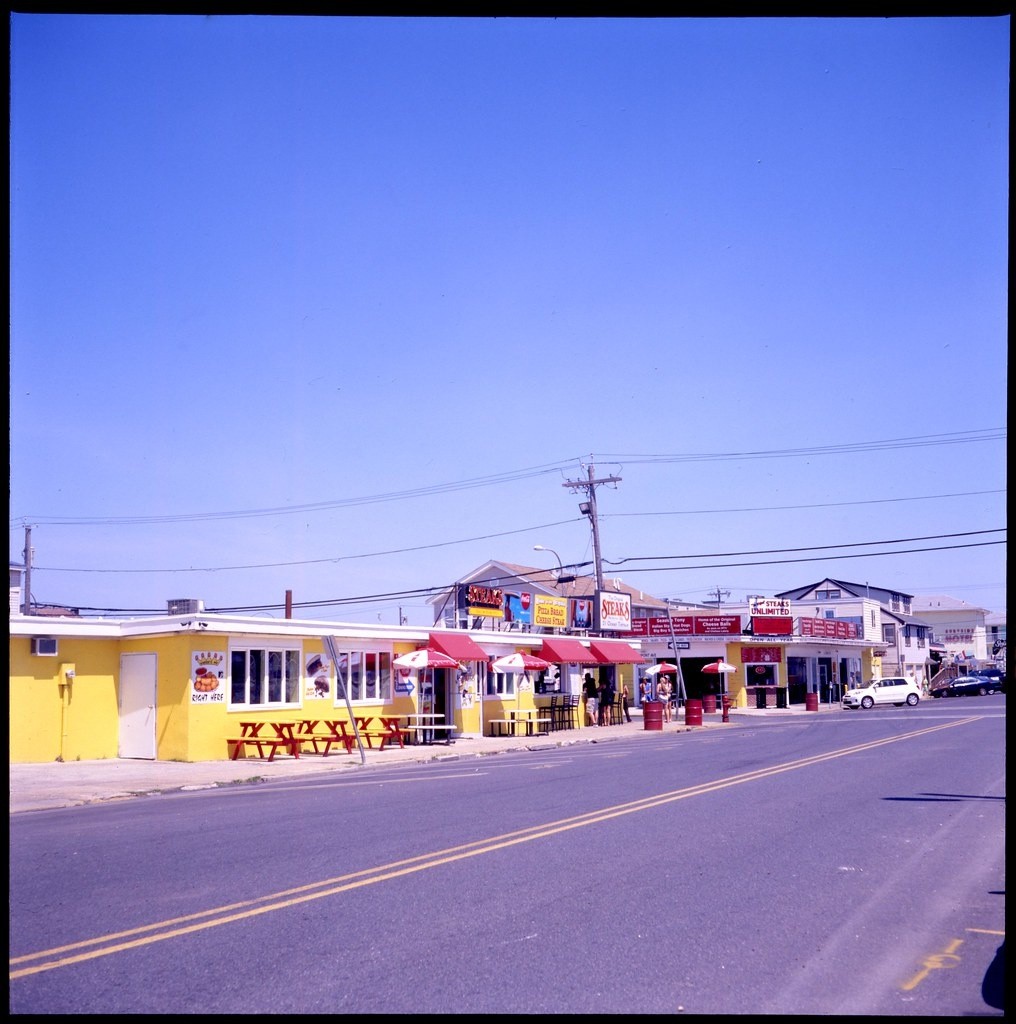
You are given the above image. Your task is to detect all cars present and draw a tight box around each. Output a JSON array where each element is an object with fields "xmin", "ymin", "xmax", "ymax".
[
  {"xmin": 842, "ymin": 677, "xmax": 923, "ymax": 709},
  {"xmin": 932, "ymin": 677, "xmax": 992, "ymax": 699}
]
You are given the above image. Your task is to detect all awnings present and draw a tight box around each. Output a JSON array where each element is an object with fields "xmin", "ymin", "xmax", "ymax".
[
  {"xmin": 590, "ymin": 642, "xmax": 646, "ymax": 664},
  {"xmin": 925, "ymin": 657, "xmax": 937, "ymax": 666},
  {"xmin": 429, "ymin": 632, "xmax": 490, "ymax": 663},
  {"xmin": 531, "ymin": 639, "xmax": 597, "ymax": 664}
]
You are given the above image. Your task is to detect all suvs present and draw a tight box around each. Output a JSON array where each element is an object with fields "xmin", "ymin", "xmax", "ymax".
[{"xmin": 968, "ymin": 668, "xmax": 1006, "ymax": 695}]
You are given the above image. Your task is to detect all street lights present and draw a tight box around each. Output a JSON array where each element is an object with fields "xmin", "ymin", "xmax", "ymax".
[{"xmin": 532, "ymin": 545, "xmax": 565, "ymax": 598}]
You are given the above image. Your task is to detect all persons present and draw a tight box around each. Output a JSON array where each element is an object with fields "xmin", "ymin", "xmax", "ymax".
[
  {"xmin": 639, "ymin": 675, "xmax": 673, "ymax": 723},
  {"xmin": 922, "ymin": 676, "xmax": 928, "ymax": 696},
  {"xmin": 583, "ymin": 673, "xmax": 632, "ymax": 728},
  {"xmin": 535, "ymin": 674, "xmax": 546, "ymax": 694}
]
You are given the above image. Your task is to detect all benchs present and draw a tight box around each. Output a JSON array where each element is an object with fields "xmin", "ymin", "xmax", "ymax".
[
  {"xmin": 227, "ymin": 725, "xmax": 457, "ymax": 762},
  {"xmin": 525, "ymin": 718, "xmax": 552, "ymax": 736},
  {"xmin": 488, "ymin": 719, "xmax": 517, "ymax": 737}
]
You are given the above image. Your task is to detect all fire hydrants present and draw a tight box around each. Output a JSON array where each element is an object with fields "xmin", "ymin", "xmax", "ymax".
[{"xmin": 722, "ymin": 695, "xmax": 732, "ymax": 722}]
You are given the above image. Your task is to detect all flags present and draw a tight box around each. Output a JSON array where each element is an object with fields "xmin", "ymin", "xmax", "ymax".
[{"xmin": 954, "ymin": 650, "xmax": 966, "ymax": 663}]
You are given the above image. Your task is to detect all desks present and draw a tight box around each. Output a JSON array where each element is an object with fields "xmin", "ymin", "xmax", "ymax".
[
  {"xmin": 290, "ymin": 718, "xmax": 352, "ymax": 757},
  {"xmin": 399, "ymin": 714, "xmax": 444, "ymax": 745},
  {"xmin": 232, "ymin": 720, "xmax": 303, "ymax": 762},
  {"xmin": 343, "ymin": 714, "xmax": 407, "ymax": 751},
  {"xmin": 498, "ymin": 709, "xmax": 539, "ymax": 736}
]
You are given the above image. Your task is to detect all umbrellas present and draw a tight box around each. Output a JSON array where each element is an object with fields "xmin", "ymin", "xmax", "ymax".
[
  {"xmin": 488, "ymin": 650, "xmax": 552, "ymax": 736},
  {"xmin": 392, "ymin": 649, "xmax": 457, "ymax": 745},
  {"xmin": 646, "ymin": 661, "xmax": 677, "ymax": 678},
  {"xmin": 702, "ymin": 660, "xmax": 737, "ymax": 709}
]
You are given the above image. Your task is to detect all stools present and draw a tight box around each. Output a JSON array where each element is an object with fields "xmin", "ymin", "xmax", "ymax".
[
  {"xmin": 539, "ymin": 695, "xmax": 580, "ymax": 734},
  {"xmin": 589, "ymin": 692, "xmax": 623, "ymax": 727}
]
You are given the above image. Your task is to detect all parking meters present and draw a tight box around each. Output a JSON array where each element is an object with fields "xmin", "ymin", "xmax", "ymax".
[{"xmin": 829, "ymin": 681, "xmax": 833, "ymax": 708}]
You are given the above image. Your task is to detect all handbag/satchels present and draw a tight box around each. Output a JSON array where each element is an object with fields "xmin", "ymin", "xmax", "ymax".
[
  {"xmin": 641, "ymin": 694, "xmax": 647, "ymax": 701},
  {"xmin": 658, "ymin": 694, "xmax": 668, "ymax": 704}
]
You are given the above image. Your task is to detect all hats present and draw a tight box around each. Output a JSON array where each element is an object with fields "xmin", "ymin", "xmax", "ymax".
[{"xmin": 665, "ymin": 675, "xmax": 670, "ymax": 680}]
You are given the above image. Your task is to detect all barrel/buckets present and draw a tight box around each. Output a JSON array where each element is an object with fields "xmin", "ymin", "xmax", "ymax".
[
  {"xmin": 806, "ymin": 693, "xmax": 818, "ymax": 711},
  {"xmin": 643, "ymin": 702, "xmax": 663, "ymax": 730},
  {"xmin": 703, "ymin": 695, "xmax": 716, "ymax": 713},
  {"xmin": 685, "ymin": 699, "xmax": 702, "ymax": 726}
]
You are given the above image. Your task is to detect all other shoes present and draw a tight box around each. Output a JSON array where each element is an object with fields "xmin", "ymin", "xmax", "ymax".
[{"xmin": 592, "ymin": 724, "xmax": 598, "ymax": 728}]
